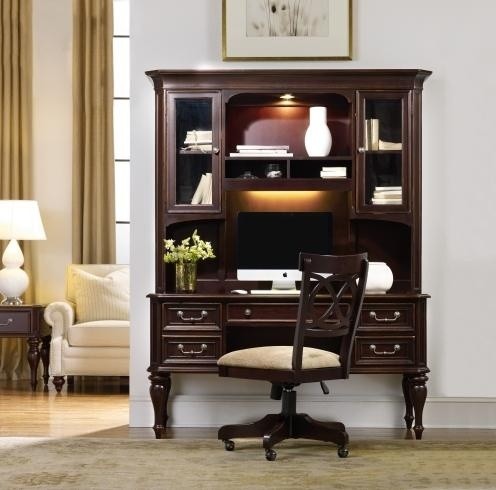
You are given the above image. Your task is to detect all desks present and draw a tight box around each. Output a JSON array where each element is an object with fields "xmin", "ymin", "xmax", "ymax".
[{"xmin": 0, "ymin": 305, "xmax": 52, "ymax": 391}]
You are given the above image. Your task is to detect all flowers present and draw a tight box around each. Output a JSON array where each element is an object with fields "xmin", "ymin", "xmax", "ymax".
[{"xmin": 163, "ymin": 228, "xmax": 217, "ymax": 263}]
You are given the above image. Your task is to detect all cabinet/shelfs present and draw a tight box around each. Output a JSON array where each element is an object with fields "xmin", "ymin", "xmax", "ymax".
[{"xmin": 144, "ymin": 68, "xmax": 433, "ymax": 439}]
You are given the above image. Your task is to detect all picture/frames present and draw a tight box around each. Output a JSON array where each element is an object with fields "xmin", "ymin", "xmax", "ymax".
[{"xmin": 220, "ymin": 0, "xmax": 355, "ymax": 63}]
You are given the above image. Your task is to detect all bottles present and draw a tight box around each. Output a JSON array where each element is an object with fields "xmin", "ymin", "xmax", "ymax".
[{"xmin": 356, "ymin": 261, "xmax": 393, "ymax": 294}]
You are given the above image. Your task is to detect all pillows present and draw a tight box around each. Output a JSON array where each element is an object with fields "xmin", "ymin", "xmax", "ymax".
[{"xmin": 71, "ymin": 266, "xmax": 131, "ymax": 325}]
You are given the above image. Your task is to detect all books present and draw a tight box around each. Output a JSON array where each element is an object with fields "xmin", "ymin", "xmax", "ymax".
[
  {"xmin": 364, "ymin": 118, "xmax": 402, "ymax": 150},
  {"xmin": 229, "ymin": 145, "xmax": 293, "ymax": 157},
  {"xmin": 320, "ymin": 166, "xmax": 348, "ymax": 179},
  {"xmin": 191, "ymin": 172, "xmax": 212, "ymax": 204},
  {"xmin": 371, "ymin": 186, "xmax": 402, "ymax": 205},
  {"xmin": 180, "ymin": 130, "xmax": 212, "ymax": 151}
]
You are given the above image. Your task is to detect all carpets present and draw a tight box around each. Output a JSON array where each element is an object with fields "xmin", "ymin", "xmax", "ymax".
[{"xmin": 0, "ymin": 425, "xmax": 496, "ymax": 490}]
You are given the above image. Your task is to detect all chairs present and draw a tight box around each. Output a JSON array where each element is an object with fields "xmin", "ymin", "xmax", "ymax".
[
  {"xmin": 216, "ymin": 251, "xmax": 370, "ymax": 462},
  {"xmin": 43, "ymin": 263, "xmax": 131, "ymax": 394}
]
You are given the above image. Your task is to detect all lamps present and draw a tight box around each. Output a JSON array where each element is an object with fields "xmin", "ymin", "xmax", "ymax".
[{"xmin": 0, "ymin": 199, "xmax": 47, "ymax": 305}]
